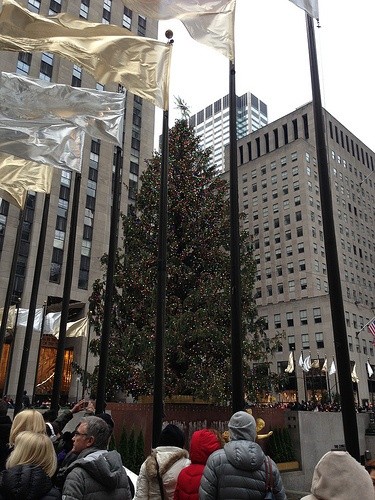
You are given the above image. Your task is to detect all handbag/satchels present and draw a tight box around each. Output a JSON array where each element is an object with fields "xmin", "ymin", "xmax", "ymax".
[{"xmin": 264, "ymin": 455, "xmax": 277, "ymax": 499}]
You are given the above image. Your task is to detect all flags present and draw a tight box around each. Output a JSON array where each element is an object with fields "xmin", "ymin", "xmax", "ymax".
[
  {"xmin": 0, "ymin": 0, "xmax": 174, "ymax": 111},
  {"xmin": 123, "ymin": 0, "xmax": 236, "ymax": 65},
  {"xmin": 0, "ymin": 71, "xmax": 126, "ymax": 210},
  {"xmin": 0, "ymin": 305, "xmax": 89, "ymax": 340},
  {"xmin": 368, "ymin": 320, "xmax": 375, "ymax": 337},
  {"xmin": 288, "ymin": 0, "xmax": 319, "ymax": 28},
  {"xmin": 285, "ymin": 351, "xmax": 373, "ymax": 383}
]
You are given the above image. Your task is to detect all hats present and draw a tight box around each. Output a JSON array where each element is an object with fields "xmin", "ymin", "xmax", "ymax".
[
  {"xmin": 228, "ymin": 411, "xmax": 256, "ymax": 442},
  {"xmin": 160, "ymin": 424, "xmax": 184, "ymax": 449}
]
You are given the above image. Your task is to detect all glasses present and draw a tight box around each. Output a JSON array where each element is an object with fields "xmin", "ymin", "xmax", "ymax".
[{"xmin": 74, "ymin": 431, "xmax": 89, "ymax": 437}]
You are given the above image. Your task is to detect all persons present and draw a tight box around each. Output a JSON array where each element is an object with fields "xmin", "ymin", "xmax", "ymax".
[
  {"xmin": 261, "ymin": 389, "xmax": 375, "ymax": 413},
  {"xmin": 173, "ymin": 428, "xmax": 226, "ymax": 500},
  {"xmin": 133, "ymin": 424, "xmax": 191, "ymax": 500},
  {"xmin": 0, "ymin": 390, "xmax": 131, "ymax": 500},
  {"xmin": 301, "ymin": 451, "xmax": 375, "ymax": 500},
  {"xmin": 198, "ymin": 411, "xmax": 287, "ymax": 500}
]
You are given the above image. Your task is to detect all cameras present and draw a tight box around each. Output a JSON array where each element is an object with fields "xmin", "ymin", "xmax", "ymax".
[{"xmin": 81, "ymin": 402, "xmax": 89, "ymax": 408}]
